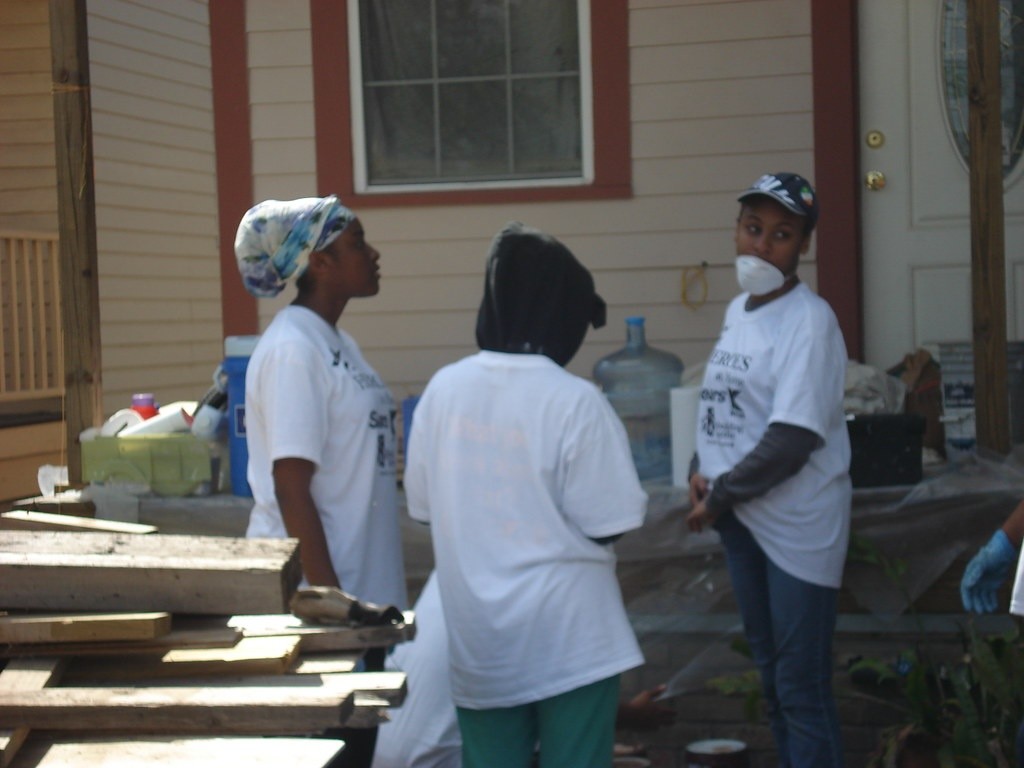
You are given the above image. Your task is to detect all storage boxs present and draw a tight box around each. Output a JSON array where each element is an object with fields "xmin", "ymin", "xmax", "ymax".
[{"xmin": 80, "ymin": 433, "xmax": 212, "ymax": 499}]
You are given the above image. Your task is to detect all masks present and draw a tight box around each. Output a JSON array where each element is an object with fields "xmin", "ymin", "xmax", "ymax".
[{"xmin": 735, "ymin": 255, "xmax": 797, "ymax": 295}]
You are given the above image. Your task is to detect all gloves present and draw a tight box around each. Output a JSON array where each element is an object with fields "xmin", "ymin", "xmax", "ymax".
[{"xmin": 962, "ymin": 529, "xmax": 1020, "ymax": 614}]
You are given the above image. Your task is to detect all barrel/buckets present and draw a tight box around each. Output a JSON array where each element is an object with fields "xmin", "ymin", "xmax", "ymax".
[
  {"xmin": 938, "ymin": 340, "xmax": 1024, "ymax": 471},
  {"xmin": 212, "ymin": 336, "xmax": 260, "ymax": 499}
]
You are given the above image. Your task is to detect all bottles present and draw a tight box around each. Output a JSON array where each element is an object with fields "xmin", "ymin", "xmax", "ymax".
[
  {"xmin": 130, "ymin": 394, "xmax": 160, "ymax": 419},
  {"xmin": 592, "ymin": 316, "xmax": 684, "ymax": 482}
]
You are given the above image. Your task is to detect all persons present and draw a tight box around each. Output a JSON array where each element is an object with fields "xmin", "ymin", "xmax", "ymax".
[
  {"xmin": 404, "ymin": 224, "xmax": 649, "ymax": 768},
  {"xmin": 236, "ymin": 197, "xmax": 410, "ymax": 768},
  {"xmin": 959, "ymin": 501, "xmax": 1024, "ymax": 615},
  {"xmin": 373, "ymin": 566, "xmax": 679, "ymax": 768},
  {"xmin": 687, "ymin": 172, "xmax": 852, "ymax": 768}
]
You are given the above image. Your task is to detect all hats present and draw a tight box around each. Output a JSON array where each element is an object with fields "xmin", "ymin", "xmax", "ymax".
[{"xmin": 737, "ymin": 171, "xmax": 818, "ymax": 231}]
